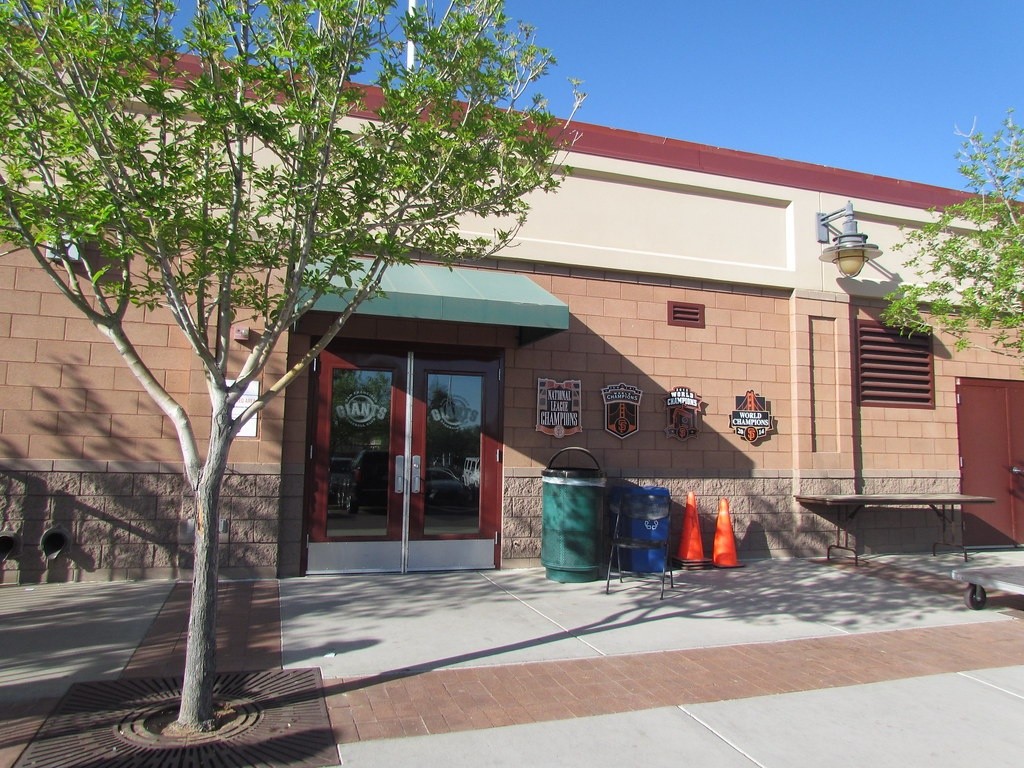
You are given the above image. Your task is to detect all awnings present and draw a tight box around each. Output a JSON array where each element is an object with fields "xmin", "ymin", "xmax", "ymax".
[{"xmin": 291, "ymin": 251, "xmax": 571, "ymax": 350}]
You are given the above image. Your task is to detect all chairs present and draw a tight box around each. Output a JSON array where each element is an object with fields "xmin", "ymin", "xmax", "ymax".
[{"xmin": 603, "ymin": 493, "xmax": 672, "ymax": 599}]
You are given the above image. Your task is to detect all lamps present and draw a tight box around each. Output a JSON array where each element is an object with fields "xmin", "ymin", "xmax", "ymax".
[{"xmin": 817, "ymin": 202, "xmax": 882, "ymax": 277}]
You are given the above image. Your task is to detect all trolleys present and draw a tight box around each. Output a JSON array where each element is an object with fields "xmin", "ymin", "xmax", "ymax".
[{"xmin": 950, "ymin": 563, "xmax": 1023, "ymax": 611}]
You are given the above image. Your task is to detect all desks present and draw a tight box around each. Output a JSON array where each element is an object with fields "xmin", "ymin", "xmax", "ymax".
[{"xmin": 792, "ymin": 493, "xmax": 997, "ymax": 567}]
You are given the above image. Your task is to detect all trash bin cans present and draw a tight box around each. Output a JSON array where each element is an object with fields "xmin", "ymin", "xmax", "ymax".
[
  {"xmin": 540, "ymin": 447, "xmax": 608, "ymax": 583},
  {"xmin": 610, "ymin": 485, "xmax": 671, "ymax": 572}
]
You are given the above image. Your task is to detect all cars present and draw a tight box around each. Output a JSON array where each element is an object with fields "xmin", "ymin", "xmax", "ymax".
[{"xmin": 329, "ymin": 449, "xmax": 480, "ymax": 515}]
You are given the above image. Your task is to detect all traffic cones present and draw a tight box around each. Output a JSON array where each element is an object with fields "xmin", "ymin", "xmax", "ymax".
[
  {"xmin": 710, "ymin": 498, "xmax": 745, "ymax": 569},
  {"xmin": 672, "ymin": 491, "xmax": 714, "ymax": 571}
]
